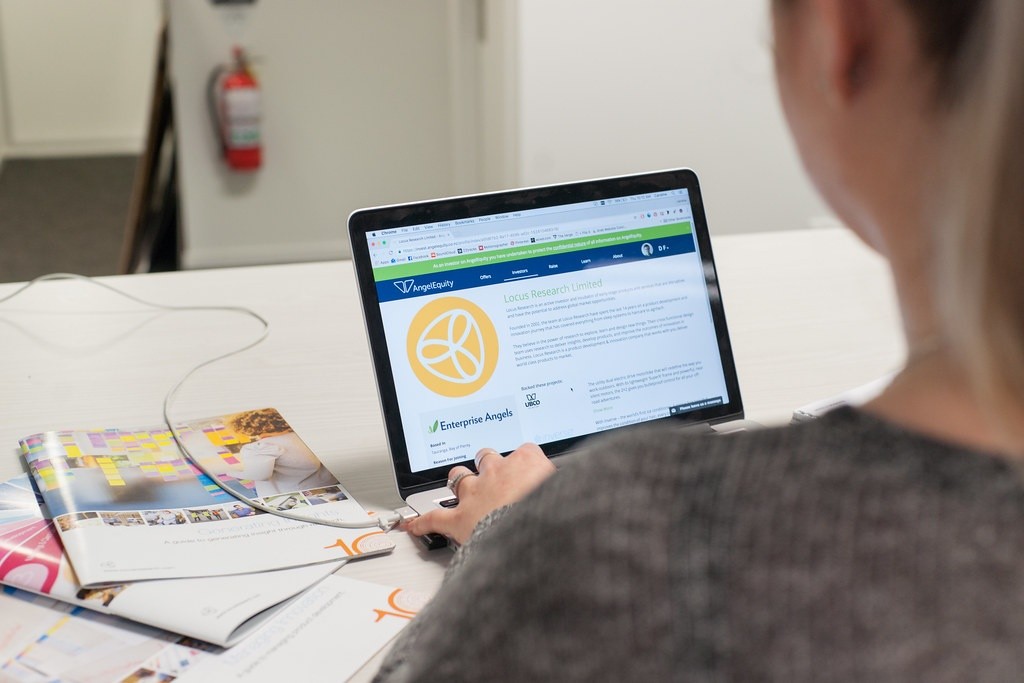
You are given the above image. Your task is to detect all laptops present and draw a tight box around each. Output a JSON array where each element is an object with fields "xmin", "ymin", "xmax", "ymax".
[{"xmin": 349, "ymin": 168, "xmax": 767, "ymax": 553}]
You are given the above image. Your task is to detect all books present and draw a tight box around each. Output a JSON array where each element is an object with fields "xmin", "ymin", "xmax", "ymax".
[{"xmin": 0, "ymin": 407, "xmax": 428, "ymax": 683}]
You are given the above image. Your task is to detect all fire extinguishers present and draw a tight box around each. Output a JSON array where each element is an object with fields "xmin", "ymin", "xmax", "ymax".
[{"xmin": 204, "ymin": 43, "xmax": 266, "ymax": 171}]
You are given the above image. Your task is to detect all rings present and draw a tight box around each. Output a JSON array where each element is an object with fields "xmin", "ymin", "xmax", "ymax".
[
  {"xmin": 446, "ymin": 471, "xmax": 475, "ymax": 495},
  {"xmin": 476, "ymin": 451, "xmax": 497, "ymax": 471}
]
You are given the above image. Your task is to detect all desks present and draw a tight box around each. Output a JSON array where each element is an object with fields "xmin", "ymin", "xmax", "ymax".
[{"xmin": 0, "ymin": 224, "xmax": 911, "ymax": 683}]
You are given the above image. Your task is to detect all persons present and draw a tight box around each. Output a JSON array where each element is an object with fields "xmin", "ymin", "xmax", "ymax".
[
  {"xmin": 175, "ymin": 512, "xmax": 186, "ymax": 524},
  {"xmin": 246, "ymin": 500, "xmax": 269, "ymax": 516},
  {"xmin": 201, "ymin": 510, "xmax": 212, "ymax": 520},
  {"xmin": 230, "ymin": 504, "xmax": 251, "ymax": 519},
  {"xmin": 191, "ymin": 512, "xmax": 200, "ymax": 521},
  {"xmin": 157, "ymin": 511, "xmax": 175, "ymax": 525},
  {"xmin": 212, "ymin": 510, "xmax": 222, "ymax": 520},
  {"xmin": 370, "ymin": 0, "xmax": 1024, "ymax": 683},
  {"xmin": 227, "ymin": 409, "xmax": 339, "ymax": 499}
]
